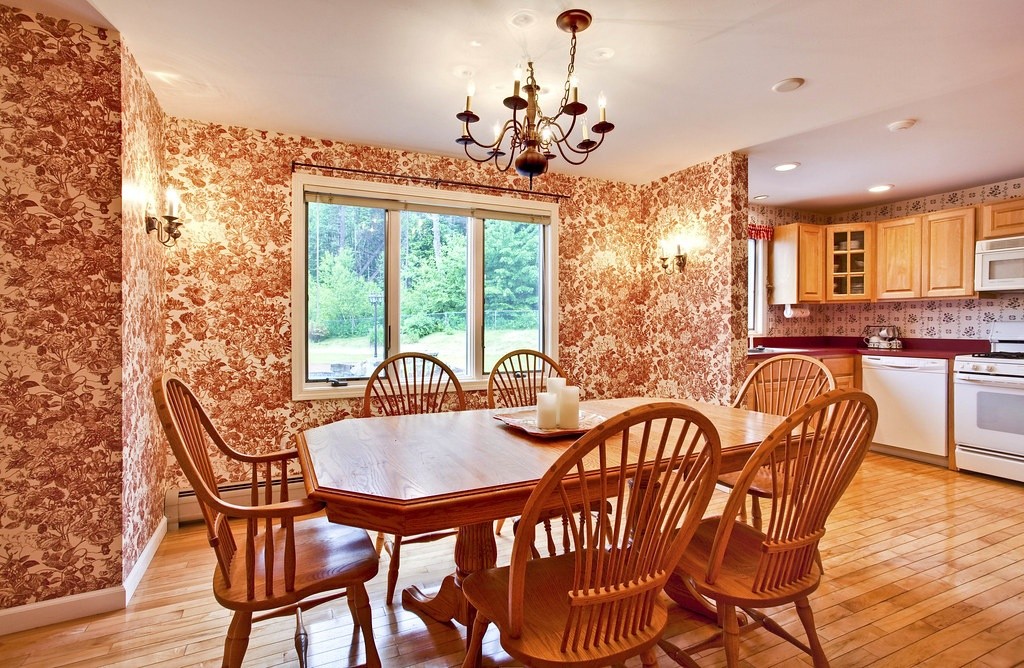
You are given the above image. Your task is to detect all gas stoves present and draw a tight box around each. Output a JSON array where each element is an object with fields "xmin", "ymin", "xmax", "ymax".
[{"xmin": 954, "ymin": 320, "xmax": 1024, "ymax": 381}]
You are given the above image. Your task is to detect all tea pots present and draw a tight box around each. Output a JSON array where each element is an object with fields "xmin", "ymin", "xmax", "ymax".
[{"xmin": 863, "ymin": 334, "xmax": 890, "ymax": 348}]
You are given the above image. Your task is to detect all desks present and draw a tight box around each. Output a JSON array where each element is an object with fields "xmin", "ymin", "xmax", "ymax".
[{"xmin": 293, "ymin": 397, "xmax": 855, "ymax": 668}]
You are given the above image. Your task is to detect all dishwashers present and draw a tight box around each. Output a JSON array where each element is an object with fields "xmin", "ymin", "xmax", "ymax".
[{"xmin": 860, "ymin": 354, "xmax": 950, "ymax": 458}]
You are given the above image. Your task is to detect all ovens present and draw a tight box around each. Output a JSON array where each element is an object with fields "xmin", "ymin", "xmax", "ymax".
[
  {"xmin": 975, "ymin": 237, "xmax": 1024, "ymax": 294},
  {"xmin": 952, "ymin": 354, "xmax": 1024, "ymax": 459}
]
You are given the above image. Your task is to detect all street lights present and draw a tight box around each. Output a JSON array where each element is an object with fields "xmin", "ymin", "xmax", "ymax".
[{"xmin": 369, "ymin": 291, "xmax": 382, "ymax": 358}]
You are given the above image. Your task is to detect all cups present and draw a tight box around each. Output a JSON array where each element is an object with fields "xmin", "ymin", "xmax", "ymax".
[{"xmin": 878, "ymin": 329, "xmax": 902, "ymax": 349}]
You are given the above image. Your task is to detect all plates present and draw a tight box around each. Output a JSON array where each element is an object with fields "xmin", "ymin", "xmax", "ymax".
[{"xmin": 833, "ymin": 246, "xmax": 865, "ymax": 290}]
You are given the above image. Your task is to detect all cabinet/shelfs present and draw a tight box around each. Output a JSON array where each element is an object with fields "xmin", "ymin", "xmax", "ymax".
[
  {"xmin": 747, "ymin": 355, "xmax": 855, "ymax": 467},
  {"xmin": 768, "ymin": 196, "xmax": 1024, "ymax": 306}
]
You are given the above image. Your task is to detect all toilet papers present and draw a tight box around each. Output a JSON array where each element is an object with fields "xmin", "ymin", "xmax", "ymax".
[{"xmin": 783, "ymin": 308, "xmax": 810, "ymax": 318}]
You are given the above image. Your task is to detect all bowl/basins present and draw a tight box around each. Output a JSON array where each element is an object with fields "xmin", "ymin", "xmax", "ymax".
[{"xmin": 839, "ymin": 240, "xmax": 860, "ymax": 250}]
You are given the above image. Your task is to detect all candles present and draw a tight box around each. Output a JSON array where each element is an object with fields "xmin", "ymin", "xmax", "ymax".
[
  {"xmin": 536, "ymin": 392, "xmax": 558, "ymax": 429},
  {"xmin": 558, "ymin": 386, "xmax": 580, "ymax": 429},
  {"xmin": 547, "ymin": 377, "xmax": 566, "ymax": 424}
]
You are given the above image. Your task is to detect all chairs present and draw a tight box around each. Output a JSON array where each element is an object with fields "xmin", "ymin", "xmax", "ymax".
[
  {"xmin": 638, "ymin": 387, "xmax": 880, "ymax": 668},
  {"xmin": 486, "ymin": 349, "xmax": 615, "ymax": 534},
  {"xmin": 364, "ymin": 352, "xmax": 464, "ymax": 607},
  {"xmin": 460, "ymin": 402, "xmax": 723, "ymax": 668},
  {"xmin": 152, "ymin": 372, "xmax": 383, "ymax": 668},
  {"xmin": 684, "ymin": 354, "xmax": 839, "ymax": 576}
]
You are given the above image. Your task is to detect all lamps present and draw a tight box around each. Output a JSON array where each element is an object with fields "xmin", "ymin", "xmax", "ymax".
[
  {"xmin": 659, "ymin": 242, "xmax": 687, "ymax": 275},
  {"xmin": 455, "ymin": 8, "xmax": 616, "ymax": 193},
  {"xmin": 144, "ymin": 186, "xmax": 184, "ymax": 248}
]
